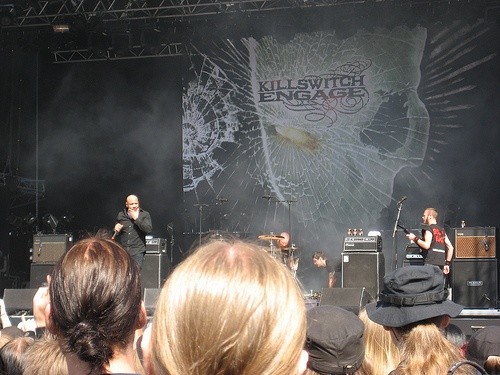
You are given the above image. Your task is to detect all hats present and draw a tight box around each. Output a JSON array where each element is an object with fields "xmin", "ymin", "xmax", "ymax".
[
  {"xmin": 304, "ymin": 305, "xmax": 365, "ymax": 375},
  {"xmin": 466, "ymin": 326, "xmax": 500, "ymax": 370},
  {"xmin": 366, "ymin": 265, "xmax": 463, "ymax": 327}
]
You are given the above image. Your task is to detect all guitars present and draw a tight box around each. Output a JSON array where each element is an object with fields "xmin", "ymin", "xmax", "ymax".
[{"xmin": 397, "ymin": 223, "xmax": 423, "ymax": 256}]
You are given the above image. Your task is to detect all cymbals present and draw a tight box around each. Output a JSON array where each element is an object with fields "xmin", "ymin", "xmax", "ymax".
[
  {"xmin": 283, "ymin": 247, "xmax": 300, "ymax": 252},
  {"xmin": 258, "ymin": 235, "xmax": 284, "ymax": 240}
]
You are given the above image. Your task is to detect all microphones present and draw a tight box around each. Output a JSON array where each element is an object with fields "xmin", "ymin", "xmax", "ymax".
[
  {"xmin": 396, "ymin": 196, "xmax": 407, "ymax": 205},
  {"xmin": 112, "ymin": 231, "xmax": 117, "ymax": 239}
]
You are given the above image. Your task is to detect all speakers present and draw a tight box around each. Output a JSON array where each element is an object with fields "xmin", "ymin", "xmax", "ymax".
[
  {"xmin": 3, "ymin": 288, "xmax": 38, "ymax": 316},
  {"xmin": 340, "ymin": 252, "xmax": 385, "ymax": 303},
  {"xmin": 450, "ymin": 258, "xmax": 497, "ymax": 309},
  {"xmin": 144, "ymin": 288, "xmax": 162, "ymax": 316},
  {"xmin": 141, "ymin": 253, "xmax": 169, "ymax": 291},
  {"xmin": 454, "ymin": 226, "xmax": 496, "ymax": 259},
  {"xmin": 33, "ymin": 234, "xmax": 72, "ymax": 263},
  {"xmin": 30, "ymin": 263, "xmax": 58, "ymax": 289},
  {"xmin": 319, "ymin": 287, "xmax": 371, "ymax": 314}
]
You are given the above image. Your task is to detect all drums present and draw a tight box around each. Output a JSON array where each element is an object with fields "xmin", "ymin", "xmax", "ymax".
[{"xmin": 266, "ymin": 248, "xmax": 287, "ymax": 267}]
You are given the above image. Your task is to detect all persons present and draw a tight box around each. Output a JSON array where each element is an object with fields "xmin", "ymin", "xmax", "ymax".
[
  {"xmin": 21, "ymin": 234, "xmax": 146, "ymax": 375},
  {"xmin": 312, "ymin": 250, "xmax": 342, "ymax": 288},
  {"xmin": 146, "ymin": 240, "xmax": 309, "ymax": 375},
  {"xmin": 113, "ymin": 194, "xmax": 152, "ymax": 270},
  {"xmin": 0, "ymin": 264, "xmax": 499, "ymax": 375},
  {"xmin": 406, "ymin": 207, "xmax": 453, "ymax": 274}
]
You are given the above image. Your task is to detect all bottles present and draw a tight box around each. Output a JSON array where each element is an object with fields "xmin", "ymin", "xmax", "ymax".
[
  {"xmin": 347, "ymin": 228, "xmax": 363, "ymax": 236},
  {"xmin": 461, "ymin": 220, "xmax": 466, "ymax": 228}
]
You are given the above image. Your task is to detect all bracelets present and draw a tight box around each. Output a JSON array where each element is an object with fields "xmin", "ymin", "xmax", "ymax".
[
  {"xmin": 445, "ymin": 260, "xmax": 451, "ymax": 266},
  {"xmin": 414, "ymin": 237, "xmax": 419, "ymax": 244}
]
